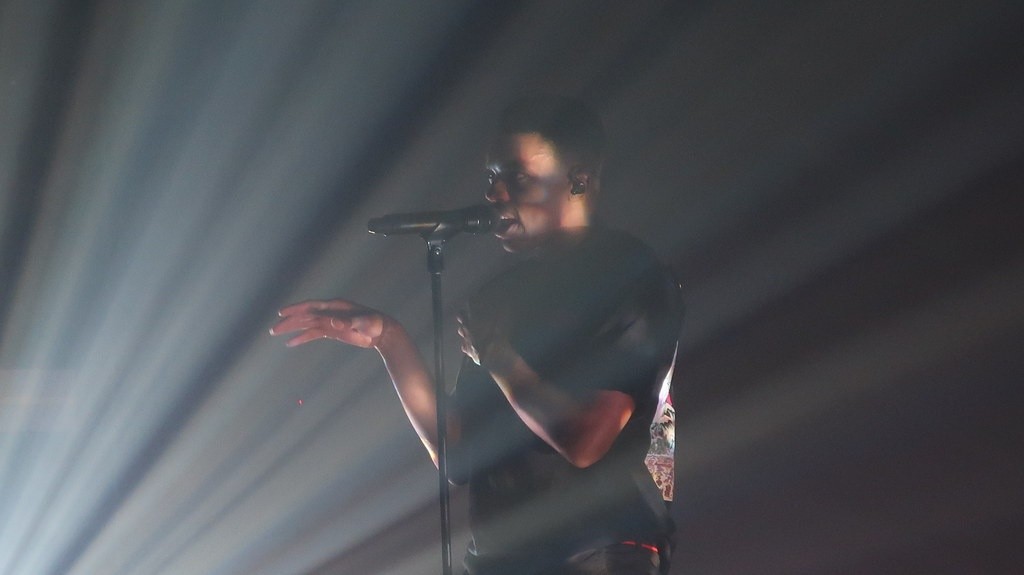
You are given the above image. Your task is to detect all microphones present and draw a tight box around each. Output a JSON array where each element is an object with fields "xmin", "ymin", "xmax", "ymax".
[{"xmin": 367, "ymin": 205, "xmax": 500, "ymax": 236}]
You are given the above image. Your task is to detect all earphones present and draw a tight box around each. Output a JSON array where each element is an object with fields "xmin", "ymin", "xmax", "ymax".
[{"xmin": 571, "ymin": 177, "xmax": 585, "ymax": 195}]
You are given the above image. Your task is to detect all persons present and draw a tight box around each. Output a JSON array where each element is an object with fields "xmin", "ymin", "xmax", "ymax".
[{"xmin": 268, "ymin": 105, "xmax": 681, "ymax": 573}]
